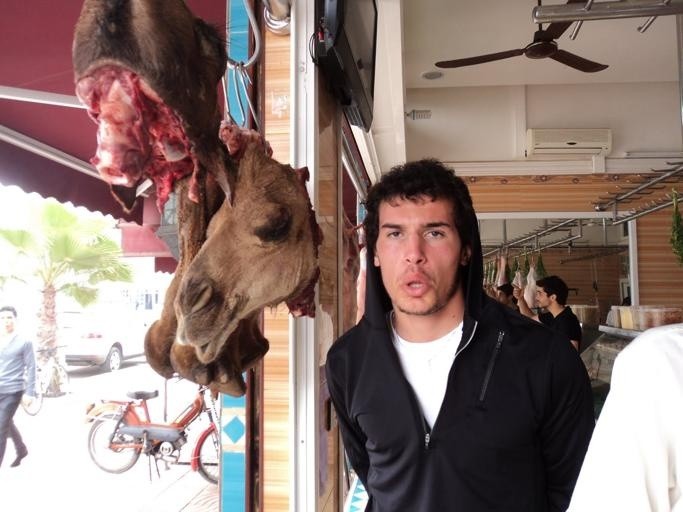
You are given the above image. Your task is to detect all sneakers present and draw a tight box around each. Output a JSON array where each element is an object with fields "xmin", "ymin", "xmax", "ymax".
[{"xmin": 11, "ymin": 449, "xmax": 30, "ymax": 469}]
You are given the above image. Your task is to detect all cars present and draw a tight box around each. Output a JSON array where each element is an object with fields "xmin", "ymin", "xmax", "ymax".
[{"xmin": 55, "ymin": 300, "xmax": 146, "ymax": 373}]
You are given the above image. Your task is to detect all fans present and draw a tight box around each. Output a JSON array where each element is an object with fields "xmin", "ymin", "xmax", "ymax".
[{"xmin": 434, "ymin": 0, "xmax": 611, "ymax": 75}]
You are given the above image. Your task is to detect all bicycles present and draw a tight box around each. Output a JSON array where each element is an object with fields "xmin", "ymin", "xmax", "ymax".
[
  {"xmin": 19, "ymin": 341, "xmax": 71, "ymax": 418},
  {"xmin": 84, "ymin": 373, "xmax": 218, "ymax": 487}
]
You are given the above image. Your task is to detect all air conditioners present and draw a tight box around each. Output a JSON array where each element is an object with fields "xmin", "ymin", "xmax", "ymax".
[{"xmin": 523, "ymin": 126, "xmax": 613, "ymax": 157}]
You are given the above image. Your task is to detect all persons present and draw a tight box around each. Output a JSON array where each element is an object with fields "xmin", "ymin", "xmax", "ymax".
[
  {"xmin": 565, "ymin": 314, "xmax": 679, "ymax": 511},
  {"xmin": 0, "ymin": 304, "xmax": 41, "ymax": 472},
  {"xmin": 495, "ymin": 284, "xmax": 519, "ymax": 316},
  {"xmin": 513, "ymin": 275, "xmax": 579, "ymax": 351},
  {"xmin": 325, "ymin": 157, "xmax": 594, "ymax": 511}
]
